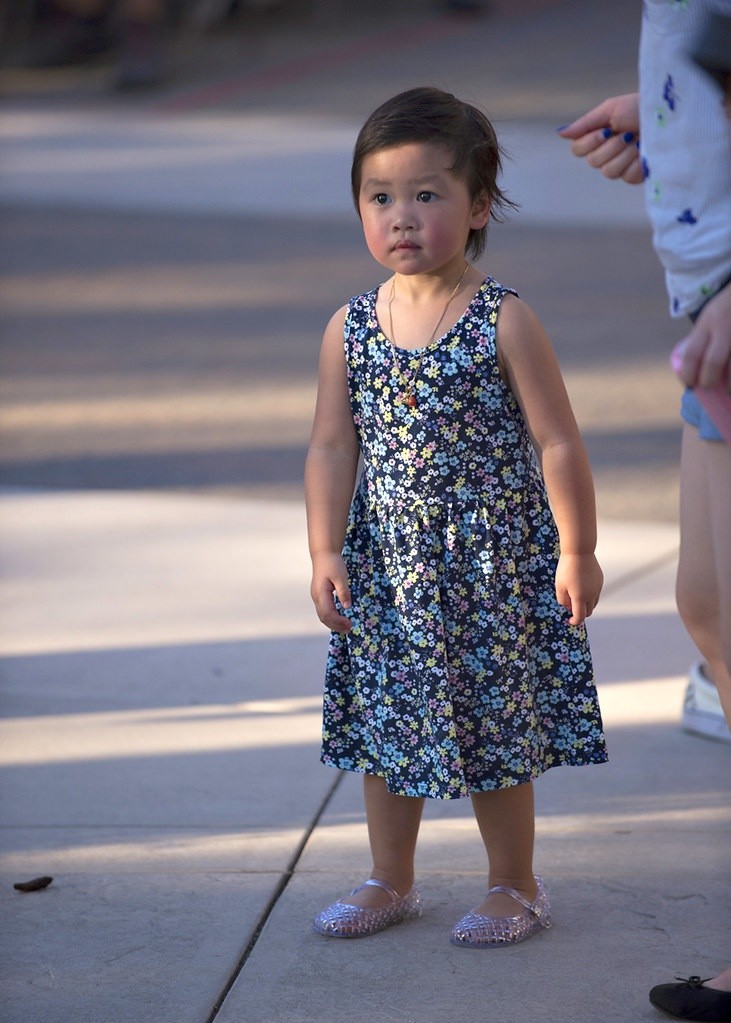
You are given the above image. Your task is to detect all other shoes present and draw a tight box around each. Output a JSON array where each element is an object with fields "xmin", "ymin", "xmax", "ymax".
[
  {"xmin": 682, "ymin": 662, "xmax": 731, "ymax": 741},
  {"xmin": 649, "ymin": 975, "xmax": 731, "ymax": 1023}
]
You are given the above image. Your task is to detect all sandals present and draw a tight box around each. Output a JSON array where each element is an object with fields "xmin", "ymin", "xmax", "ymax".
[
  {"xmin": 312, "ymin": 878, "xmax": 422, "ymax": 938},
  {"xmin": 450, "ymin": 875, "xmax": 552, "ymax": 948}
]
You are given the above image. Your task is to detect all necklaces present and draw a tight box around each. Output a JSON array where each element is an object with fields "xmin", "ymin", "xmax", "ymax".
[{"xmin": 386, "ymin": 262, "xmax": 469, "ymax": 407}]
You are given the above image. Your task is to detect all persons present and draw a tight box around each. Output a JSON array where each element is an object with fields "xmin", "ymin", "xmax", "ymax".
[
  {"xmin": 306, "ymin": 86, "xmax": 609, "ymax": 942},
  {"xmin": 562, "ymin": 0, "xmax": 731, "ymax": 1023}
]
